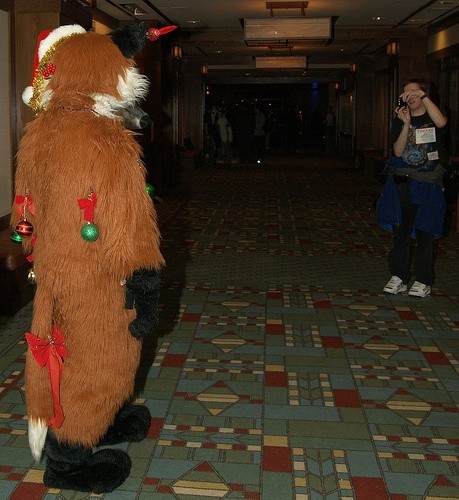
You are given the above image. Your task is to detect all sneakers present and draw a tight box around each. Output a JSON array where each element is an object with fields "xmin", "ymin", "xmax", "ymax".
[
  {"xmin": 383, "ymin": 276, "xmax": 408, "ymax": 295},
  {"xmin": 408, "ymin": 280, "xmax": 431, "ymax": 298}
]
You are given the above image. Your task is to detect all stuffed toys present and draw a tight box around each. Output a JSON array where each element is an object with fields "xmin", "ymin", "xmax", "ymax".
[{"xmin": 9, "ymin": 22, "xmax": 167, "ymax": 494}]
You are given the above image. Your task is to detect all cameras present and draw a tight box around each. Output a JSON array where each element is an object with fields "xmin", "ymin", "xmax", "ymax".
[{"xmin": 397, "ymin": 96, "xmax": 407, "ymax": 112}]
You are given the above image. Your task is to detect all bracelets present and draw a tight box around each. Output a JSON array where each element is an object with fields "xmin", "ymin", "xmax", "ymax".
[{"xmin": 419, "ymin": 92, "xmax": 430, "ymax": 100}]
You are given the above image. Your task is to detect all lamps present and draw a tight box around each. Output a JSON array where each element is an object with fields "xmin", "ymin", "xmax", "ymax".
[
  {"xmin": 242, "ymin": 17, "xmax": 334, "ymax": 49},
  {"xmin": 255, "ymin": 56, "xmax": 309, "ymax": 70},
  {"xmin": 386, "ymin": 41, "xmax": 400, "ymax": 56},
  {"xmin": 200, "ymin": 64, "xmax": 208, "ymax": 79},
  {"xmin": 171, "ymin": 45, "xmax": 182, "ymax": 59}
]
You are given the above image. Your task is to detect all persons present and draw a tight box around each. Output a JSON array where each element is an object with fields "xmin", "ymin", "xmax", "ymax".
[
  {"xmin": 292, "ymin": 106, "xmax": 344, "ymax": 157},
  {"xmin": 375, "ymin": 78, "xmax": 448, "ymax": 298},
  {"xmin": 204, "ymin": 103, "xmax": 270, "ymax": 165}
]
